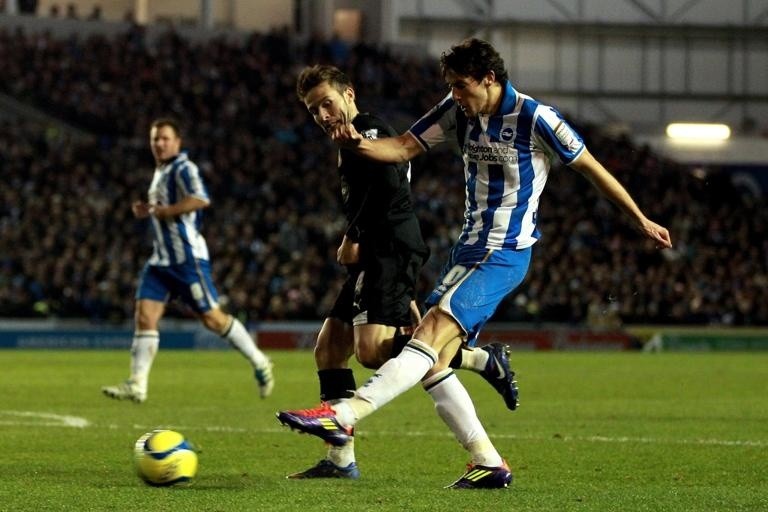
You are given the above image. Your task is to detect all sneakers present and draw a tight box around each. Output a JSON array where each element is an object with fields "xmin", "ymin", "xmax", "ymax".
[
  {"xmin": 275, "ymin": 402, "xmax": 360, "ymax": 479},
  {"xmin": 255, "ymin": 359, "xmax": 274, "ymax": 398},
  {"xmin": 102, "ymin": 379, "xmax": 147, "ymax": 403},
  {"xmin": 479, "ymin": 342, "xmax": 520, "ymax": 411},
  {"xmin": 442, "ymin": 458, "xmax": 513, "ymax": 490}
]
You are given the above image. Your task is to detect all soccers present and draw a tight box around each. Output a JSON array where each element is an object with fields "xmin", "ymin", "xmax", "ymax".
[{"xmin": 133, "ymin": 427, "xmax": 197, "ymax": 488}]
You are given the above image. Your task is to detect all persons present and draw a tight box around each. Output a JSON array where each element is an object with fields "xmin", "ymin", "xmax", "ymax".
[
  {"xmin": 1, "ymin": 18, "xmax": 767, "ymax": 328},
  {"xmin": 275, "ymin": 36, "xmax": 674, "ymax": 492},
  {"xmin": 284, "ymin": 62, "xmax": 521, "ymax": 481},
  {"xmin": 99, "ymin": 115, "xmax": 276, "ymax": 401}
]
